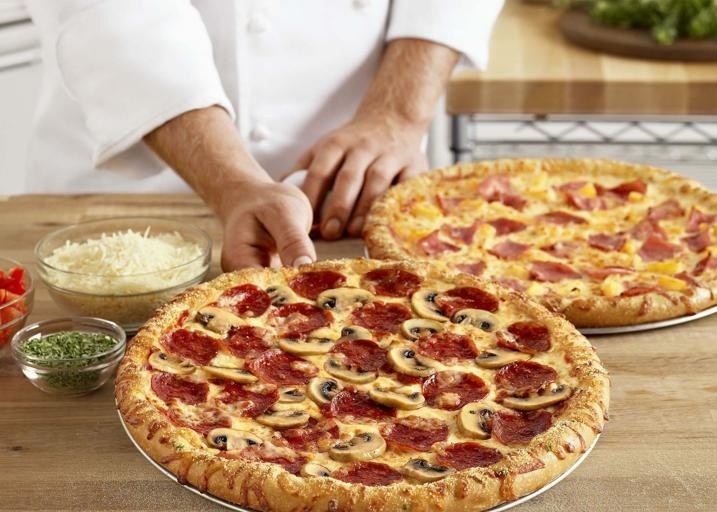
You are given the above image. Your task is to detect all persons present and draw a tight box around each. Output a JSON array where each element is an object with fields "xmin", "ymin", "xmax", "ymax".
[{"xmin": 22, "ymin": 0, "xmax": 506, "ymax": 273}]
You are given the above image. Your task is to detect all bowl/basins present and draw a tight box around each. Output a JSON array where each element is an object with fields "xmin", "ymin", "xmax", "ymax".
[
  {"xmin": 0, "ymin": 255, "xmax": 35, "ymax": 360},
  {"xmin": 9, "ymin": 315, "xmax": 127, "ymax": 399},
  {"xmin": 33, "ymin": 216, "xmax": 211, "ymax": 337}
]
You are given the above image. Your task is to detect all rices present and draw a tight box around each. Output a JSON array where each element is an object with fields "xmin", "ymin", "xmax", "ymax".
[{"xmin": 40, "ymin": 226, "xmax": 209, "ymax": 326}]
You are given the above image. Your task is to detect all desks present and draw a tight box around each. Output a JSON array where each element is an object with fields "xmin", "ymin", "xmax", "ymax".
[{"xmin": 444, "ymin": 0, "xmax": 717, "ymax": 164}]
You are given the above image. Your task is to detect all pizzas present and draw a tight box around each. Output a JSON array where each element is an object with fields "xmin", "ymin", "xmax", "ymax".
[
  {"xmin": 114, "ymin": 256, "xmax": 609, "ymax": 512},
  {"xmin": 362, "ymin": 157, "xmax": 717, "ymax": 329}
]
